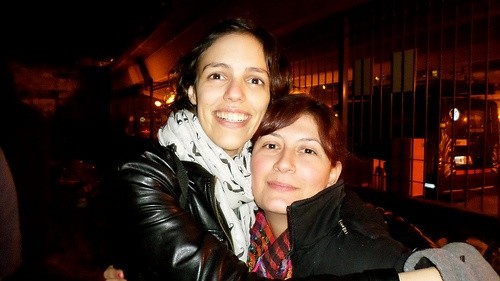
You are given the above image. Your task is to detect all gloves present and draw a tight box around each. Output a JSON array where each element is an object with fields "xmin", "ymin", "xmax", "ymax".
[{"xmin": 404, "ymin": 242, "xmax": 500, "ymax": 281}]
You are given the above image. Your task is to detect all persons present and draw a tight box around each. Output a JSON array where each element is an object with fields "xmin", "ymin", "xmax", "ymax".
[
  {"xmin": 104, "ymin": 93, "xmax": 436, "ymax": 281},
  {"xmin": 118, "ymin": 19, "xmax": 443, "ymax": 281}
]
